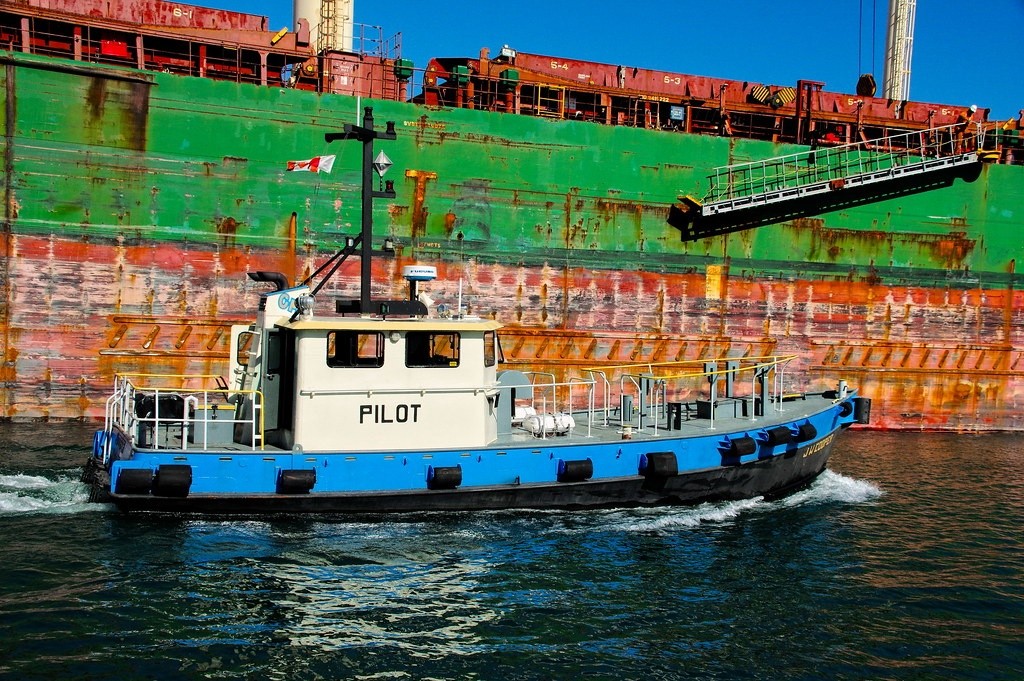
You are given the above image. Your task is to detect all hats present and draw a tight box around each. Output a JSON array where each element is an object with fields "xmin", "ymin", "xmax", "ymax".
[{"xmin": 970, "ymin": 104, "xmax": 977, "ymax": 113}]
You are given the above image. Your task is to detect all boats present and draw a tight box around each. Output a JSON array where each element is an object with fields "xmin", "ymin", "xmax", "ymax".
[{"xmin": 78, "ymin": 105, "xmax": 874, "ymax": 512}]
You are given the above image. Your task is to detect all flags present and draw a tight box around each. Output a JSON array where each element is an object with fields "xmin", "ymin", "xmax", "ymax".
[
  {"xmin": 319, "ymin": 155, "xmax": 336, "ymax": 174},
  {"xmin": 287, "ymin": 156, "xmax": 322, "ymax": 175}
]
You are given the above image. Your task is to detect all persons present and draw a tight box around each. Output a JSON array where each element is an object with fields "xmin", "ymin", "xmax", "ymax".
[
  {"xmin": 925, "ymin": 136, "xmax": 939, "ymax": 161},
  {"xmin": 824, "ymin": 125, "xmax": 844, "ymax": 148},
  {"xmin": 955, "ymin": 105, "xmax": 977, "ymax": 154}
]
